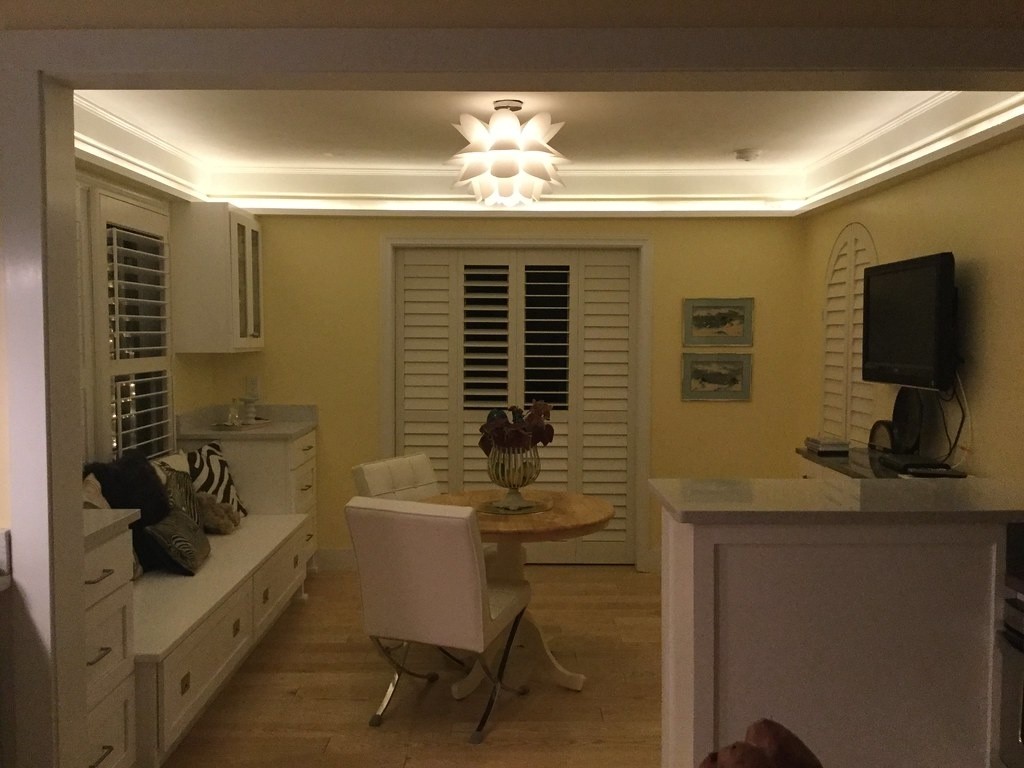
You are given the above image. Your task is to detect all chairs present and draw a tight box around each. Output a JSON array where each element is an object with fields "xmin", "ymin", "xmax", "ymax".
[{"xmin": 344, "ymin": 454, "xmax": 529, "ymax": 744}]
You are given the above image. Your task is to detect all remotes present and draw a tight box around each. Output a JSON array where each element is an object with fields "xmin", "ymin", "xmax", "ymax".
[{"xmin": 908, "ymin": 467, "xmax": 967, "ymax": 479}]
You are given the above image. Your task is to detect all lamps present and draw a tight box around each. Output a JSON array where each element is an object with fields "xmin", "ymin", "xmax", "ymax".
[{"xmin": 453, "ymin": 100, "xmax": 570, "ymax": 206}]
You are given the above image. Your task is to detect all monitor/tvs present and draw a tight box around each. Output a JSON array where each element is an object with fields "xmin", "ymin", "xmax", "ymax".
[{"xmin": 861, "ymin": 251, "xmax": 956, "ymax": 392}]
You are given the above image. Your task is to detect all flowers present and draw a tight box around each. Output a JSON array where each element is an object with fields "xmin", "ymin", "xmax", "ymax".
[{"xmin": 478, "ymin": 399, "xmax": 554, "ymax": 453}]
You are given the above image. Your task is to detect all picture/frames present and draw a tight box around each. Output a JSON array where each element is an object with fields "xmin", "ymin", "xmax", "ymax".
[
  {"xmin": 680, "ymin": 352, "xmax": 752, "ymax": 401},
  {"xmin": 683, "ymin": 296, "xmax": 754, "ymax": 347}
]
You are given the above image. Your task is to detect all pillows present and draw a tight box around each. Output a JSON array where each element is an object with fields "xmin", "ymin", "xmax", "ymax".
[{"xmin": 79, "ymin": 438, "xmax": 246, "ymax": 574}]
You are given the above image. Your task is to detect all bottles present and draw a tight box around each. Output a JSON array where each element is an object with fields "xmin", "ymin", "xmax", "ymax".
[{"xmin": 227, "ymin": 399, "xmax": 239, "ymax": 423}]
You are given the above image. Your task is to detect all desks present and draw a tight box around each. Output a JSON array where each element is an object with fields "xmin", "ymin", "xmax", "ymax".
[
  {"xmin": 649, "ymin": 448, "xmax": 1024, "ymax": 768},
  {"xmin": 425, "ymin": 490, "xmax": 614, "ymax": 700}
]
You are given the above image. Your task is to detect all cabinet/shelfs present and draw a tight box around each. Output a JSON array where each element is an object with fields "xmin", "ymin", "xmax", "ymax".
[
  {"xmin": 135, "ymin": 404, "xmax": 320, "ymax": 768},
  {"xmin": 83, "ymin": 509, "xmax": 141, "ymax": 768},
  {"xmin": 169, "ymin": 202, "xmax": 264, "ymax": 353}
]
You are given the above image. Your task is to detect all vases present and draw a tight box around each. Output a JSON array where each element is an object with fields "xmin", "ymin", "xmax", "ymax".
[{"xmin": 489, "ymin": 442, "xmax": 541, "ymax": 511}]
